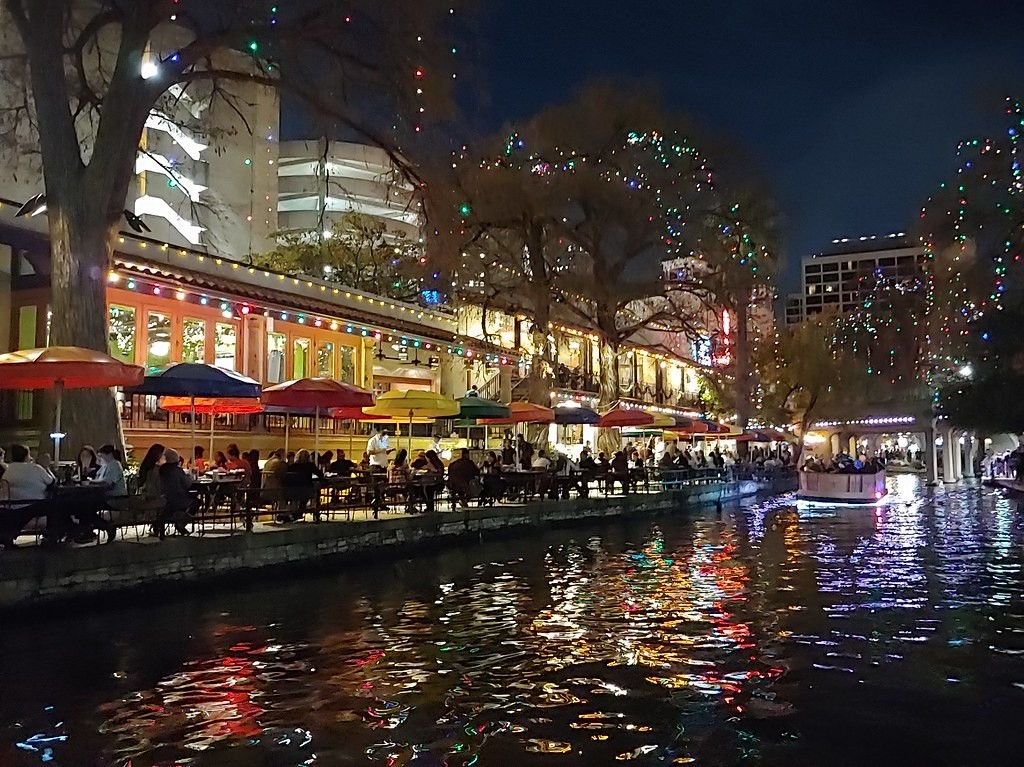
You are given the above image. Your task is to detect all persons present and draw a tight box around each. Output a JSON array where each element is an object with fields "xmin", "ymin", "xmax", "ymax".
[
  {"xmin": 1, "ymin": 429, "xmax": 1022, "ymax": 549},
  {"xmin": 551, "ymin": 358, "xmax": 728, "ymax": 416}
]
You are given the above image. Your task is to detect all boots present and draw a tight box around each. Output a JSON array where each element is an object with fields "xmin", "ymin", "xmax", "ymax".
[{"xmin": 175, "ymin": 513, "xmax": 194, "ymax": 534}]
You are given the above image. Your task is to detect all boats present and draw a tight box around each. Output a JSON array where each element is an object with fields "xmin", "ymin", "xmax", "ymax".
[
  {"xmin": 796, "ymin": 465, "xmax": 888, "ymax": 504},
  {"xmin": 981, "ymin": 473, "xmax": 1024, "ymax": 492}
]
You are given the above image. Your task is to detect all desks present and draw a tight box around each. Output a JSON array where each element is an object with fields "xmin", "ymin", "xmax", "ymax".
[
  {"xmin": 312, "ymin": 477, "xmax": 359, "ymax": 521},
  {"xmin": 412, "ymin": 474, "xmax": 425, "ymax": 478},
  {"xmin": 56, "ymin": 485, "xmax": 76, "ymax": 490},
  {"xmin": 191, "ymin": 479, "xmax": 243, "ymax": 532},
  {"xmin": 501, "ymin": 470, "xmax": 545, "ymax": 506}
]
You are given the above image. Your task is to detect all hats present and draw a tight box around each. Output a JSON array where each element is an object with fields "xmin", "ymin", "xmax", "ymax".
[
  {"xmin": 165, "ymin": 448, "xmax": 178, "ymax": 463},
  {"xmin": 805, "ymin": 456, "xmax": 814, "ymax": 463},
  {"xmin": 418, "ymin": 452, "xmax": 426, "ymax": 457},
  {"xmin": 434, "ymin": 434, "xmax": 442, "ymax": 438}
]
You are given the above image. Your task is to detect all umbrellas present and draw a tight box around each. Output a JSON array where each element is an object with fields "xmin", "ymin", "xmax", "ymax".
[{"xmin": 1, "ymin": 346, "xmax": 803, "ymax": 519}]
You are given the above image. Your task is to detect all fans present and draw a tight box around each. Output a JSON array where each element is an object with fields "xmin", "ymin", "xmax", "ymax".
[
  {"xmin": 373, "ymin": 341, "xmax": 401, "ymax": 361},
  {"xmin": 398, "ymin": 348, "xmax": 433, "ymax": 367}
]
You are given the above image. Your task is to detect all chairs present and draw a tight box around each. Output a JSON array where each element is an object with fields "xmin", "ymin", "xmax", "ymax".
[{"xmin": 0, "ymin": 464, "xmax": 798, "ymax": 549}]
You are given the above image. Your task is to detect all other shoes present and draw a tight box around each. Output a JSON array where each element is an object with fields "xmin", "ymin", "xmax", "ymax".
[
  {"xmin": 107, "ymin": 524, "xmax": 116, "ymax": 542},
  {"xmin": 424, "ymin": 507, "xmax": 434, "ymax": 512},
  {"xmin": 277, "ymin": 515, "xmax": 291, "ymax": 522},
  {"xmin": 380, "ymin": 506, "xmax": 390, "ymax": 511},
  {"xmin": 459, "ymin": 499, "xmax": 468, "ymax": 508}
]
